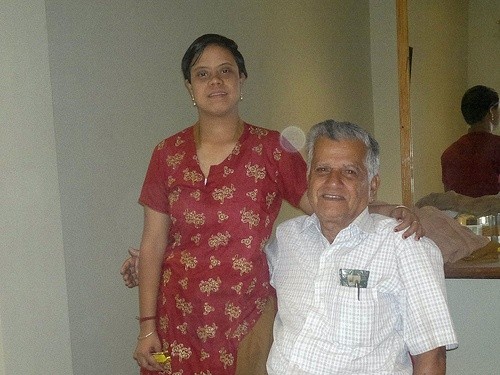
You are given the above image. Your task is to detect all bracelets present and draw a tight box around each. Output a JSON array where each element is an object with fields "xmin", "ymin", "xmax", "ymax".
[
  {"xmin": 389, "ymin": 205, "xmax": 412, "ymax": 210},
  {"xmin": 135, "ymin": 315, "xmax": 158, "ymax": 322},
  {"xmin": 136, "ymin": 329, "xmax": 157, "ymax": 340}
]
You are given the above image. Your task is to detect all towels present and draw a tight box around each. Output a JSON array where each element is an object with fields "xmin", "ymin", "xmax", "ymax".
[{"xmin": 408, "ymin": 206, "xmax": 490, "ymax": 265}]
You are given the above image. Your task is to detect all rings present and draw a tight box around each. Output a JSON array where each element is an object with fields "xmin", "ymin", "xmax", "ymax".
[{"xmin": 413, "ymin": 220, "xmax": 420, "ymax": 224}]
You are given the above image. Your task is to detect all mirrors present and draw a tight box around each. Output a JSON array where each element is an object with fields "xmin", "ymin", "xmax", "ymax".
[{"xmin": 397, "ymin": 0, "xmax": 499, "ymax": 214}]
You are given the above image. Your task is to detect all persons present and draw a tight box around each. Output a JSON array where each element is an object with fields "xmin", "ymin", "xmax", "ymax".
[
  {"xmin": 132, "ymin": 33, "xmax": 424, "ymax": 375},
  {"xmin": 346, "ymin": 271, "xmax": 363, "ymax": 288},
  {"xmin": 120, "ymin": 118, "xmax": 459, "ymax": 375},
  {"xmin": 440, "ymin": 84, "xmax": 500, "ymax": 198}
]
